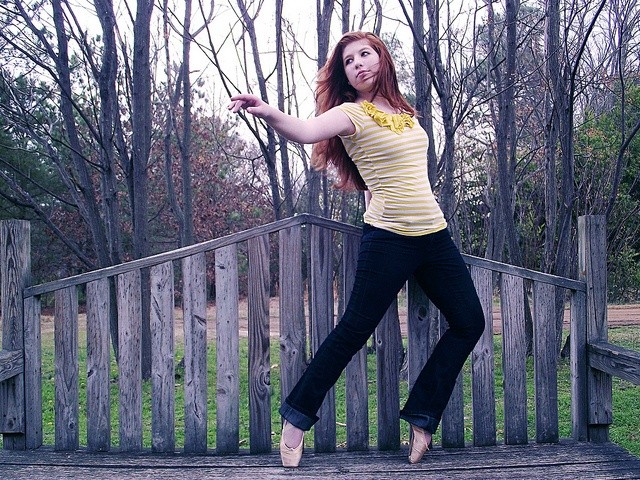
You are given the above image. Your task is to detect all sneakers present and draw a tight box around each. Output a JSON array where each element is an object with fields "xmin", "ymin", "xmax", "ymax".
[
  {"xmin": 407, "ymin": 422, "xmax": 428, "ymax": 463},
  {"xmin": 280, "ymin": 420, "xmax": 305, "ymax": 468}
]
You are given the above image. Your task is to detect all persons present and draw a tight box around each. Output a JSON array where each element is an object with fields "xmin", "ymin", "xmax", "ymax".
[{"xmin": 229, "ymin": 31, "xmax": 485, "ymax": 467}]
